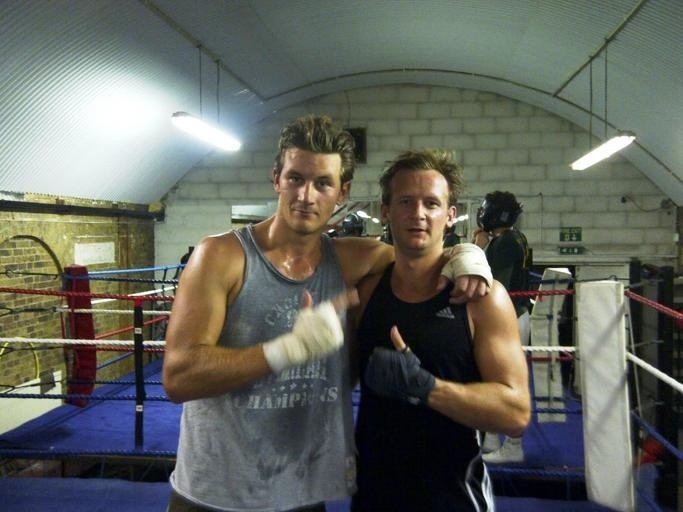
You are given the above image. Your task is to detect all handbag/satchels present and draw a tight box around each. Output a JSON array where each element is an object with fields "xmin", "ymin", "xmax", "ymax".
[{"xmin": 262, "ymin": 337, "xmax": 290, "ymax": 374}]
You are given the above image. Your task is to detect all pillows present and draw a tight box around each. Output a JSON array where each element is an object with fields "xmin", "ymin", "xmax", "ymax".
[{"xmin": 476, "ymin": 198, "xmax": 519, "ymax": 232}]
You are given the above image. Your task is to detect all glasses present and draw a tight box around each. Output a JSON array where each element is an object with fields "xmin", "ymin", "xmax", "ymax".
[{"xmin": 567, "ymin": 38, "xmax": 637, "ymax": 173}]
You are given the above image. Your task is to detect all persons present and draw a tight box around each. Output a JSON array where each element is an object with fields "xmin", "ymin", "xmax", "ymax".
[
  {"xmin": 472, "ymin": 190, "xmax": 531, "ymax": 464},
  {"xmin": 349, "ymin": 150, "xmax": 531, "ymax": 512},
  {"xmin": 333, "ymin": 212, "xmax": 364, "ymax": 237},
  {"xmin": 162, "ymin": 114, "xmax": 493, "ymax": 512},
  {"xmin": 442, "ymin": 225, "xmax": 460, "ymax": 248}
]
